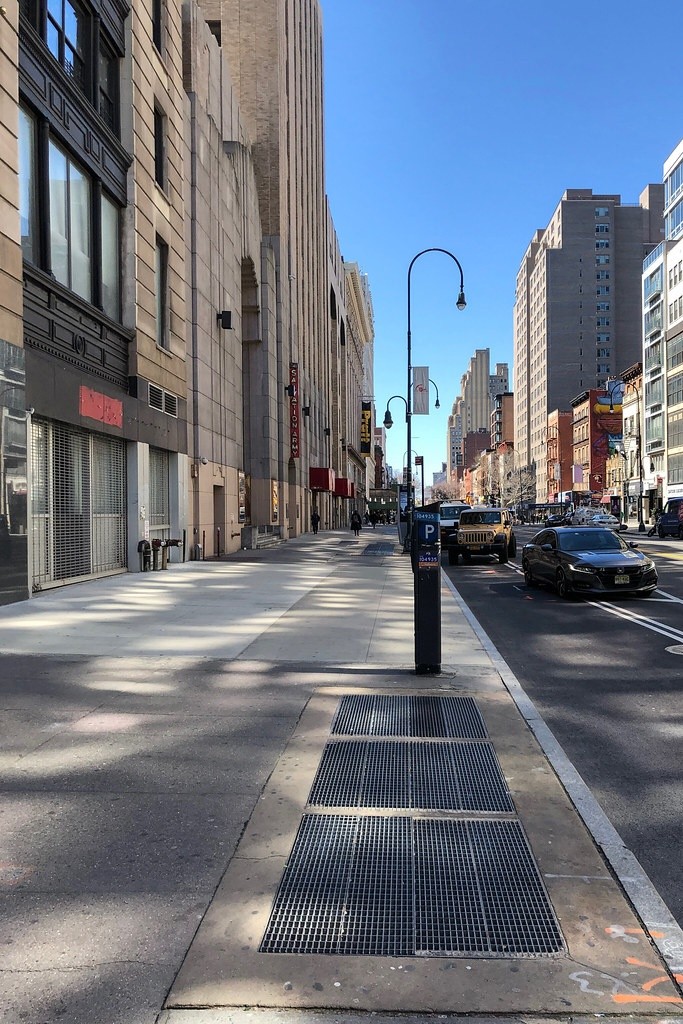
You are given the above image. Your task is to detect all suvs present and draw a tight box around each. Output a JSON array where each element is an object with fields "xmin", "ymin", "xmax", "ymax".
[{"xmin": 447, "ymin": 507, "xmax": 518, "ymax": 564}]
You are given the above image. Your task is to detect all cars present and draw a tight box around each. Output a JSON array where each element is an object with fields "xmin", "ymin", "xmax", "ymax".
[
  {"xmin": 544, "ymin": 511, "xmax": 620, "ymax": 531},
  {"xmin": 523, "ymin": 526, "xmax": 658, "ymax": 598}
]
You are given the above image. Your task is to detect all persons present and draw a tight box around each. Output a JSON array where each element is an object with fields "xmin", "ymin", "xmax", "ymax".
[
  {"xmin": 351, "ymin": 508, "xmax": 396, "ymax": 536},
  {"xmin": 311, "ymin": 510, "xmax": 320, "ymax": 534},
  {"xmin": 654, "ymin": 508, "xmax": 665, "ymax": 526},
  {"xmin": 517, "ymin": 513, "xmax": 548, "ymax": 524}
]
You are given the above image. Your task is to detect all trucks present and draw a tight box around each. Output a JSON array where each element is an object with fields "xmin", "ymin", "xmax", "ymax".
[{"xmin": 424, "ymin": 499, "xmax": 472, "ymax": 545}]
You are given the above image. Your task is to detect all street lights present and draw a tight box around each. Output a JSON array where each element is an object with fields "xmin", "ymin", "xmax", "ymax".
[
  {"xmin": 383, "ymin": 247, "xmax": 468, "ymax": 552},
  {"xmin": 402, "ymin": 450, "xmax": 419, "ymax": 482},
  {"xmin": 540, "ymin": 426, "xmax": 563, "ymax": 515},
  {"xmin": 508, "ymin": 449, "xmax": 524, "ymax": 525},
  {"xmin": 609, "ymin": 382, "xmax": 655, "ymax": 531}
]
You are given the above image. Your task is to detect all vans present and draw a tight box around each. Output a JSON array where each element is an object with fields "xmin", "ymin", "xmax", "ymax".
[{"xmin": 656, "ymin": 498, "xmax": 683, "ymax": 539}]
